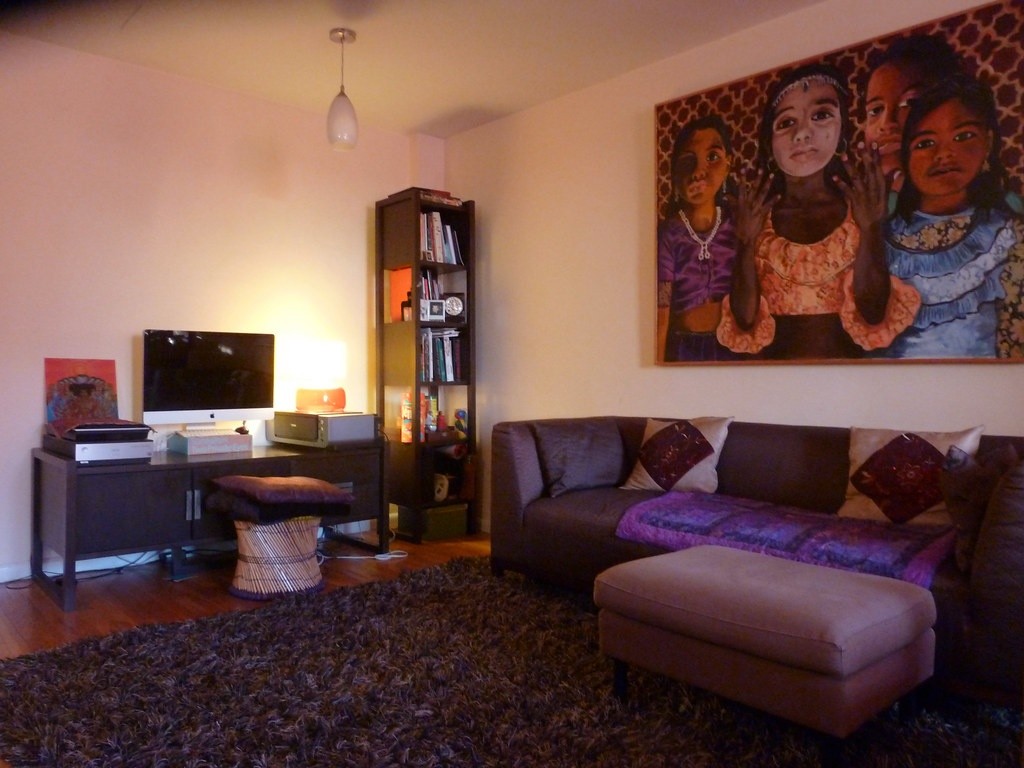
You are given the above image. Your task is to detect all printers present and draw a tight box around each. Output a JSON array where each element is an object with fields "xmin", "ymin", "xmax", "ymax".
[{"xmin": 265, "ymin": 410, "xmax": 379, "ymax": 449}]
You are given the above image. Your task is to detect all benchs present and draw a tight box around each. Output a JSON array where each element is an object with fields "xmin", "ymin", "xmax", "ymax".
[{"xmin": 595, "ymin": 545, "xmax": 939, "ymax": 759}]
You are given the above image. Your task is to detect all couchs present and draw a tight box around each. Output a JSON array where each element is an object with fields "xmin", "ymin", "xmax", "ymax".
[{"xmin": 487, "ymin": 413, "xmax": 1024, "ymax": 697}]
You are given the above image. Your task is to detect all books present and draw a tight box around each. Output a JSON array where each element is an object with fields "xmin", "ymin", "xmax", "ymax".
[{"xmin": 388, "ymin": 191, "xmax": 469, "ymax": 380}]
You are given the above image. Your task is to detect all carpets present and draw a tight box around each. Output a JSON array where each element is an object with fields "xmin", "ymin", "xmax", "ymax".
[{"xmin": 0, "ymin": 554, "xmax": 1023, "ymax": 768}]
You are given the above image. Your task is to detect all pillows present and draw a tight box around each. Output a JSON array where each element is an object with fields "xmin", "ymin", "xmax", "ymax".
[
  {"xmin": 947, "ymin": 440, "xmax": 1017, "ymax": 573},
  {"xmin": 840, "ymin": 426, "xmax": 982, "ymax": 532},
  {"xmin": 621, "ymin": 414, "xmax": 732, "ymax": 495},
  {"xmin": 529, "ymin": 420, "xmax": 629, "ymax": 499}
]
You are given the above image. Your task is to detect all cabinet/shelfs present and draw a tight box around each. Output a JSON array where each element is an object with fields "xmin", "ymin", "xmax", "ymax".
[
  {"xmin": 370, "ymin": 184, "xmax": 477, "ymax": 546},
  {"xmin": 30, "ymin": 440, "xmax": 385, "ymax": 610}
]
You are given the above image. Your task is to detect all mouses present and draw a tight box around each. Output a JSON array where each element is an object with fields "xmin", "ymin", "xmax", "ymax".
[{"xmin": 235, "ymin": 426, "xmax": 249, "ymax": 434}]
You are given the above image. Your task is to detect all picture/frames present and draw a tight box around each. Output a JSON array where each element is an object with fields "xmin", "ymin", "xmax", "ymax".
[{"xmin": 656, "ymin": 1, "xmax": 1023, "ymax": 367}]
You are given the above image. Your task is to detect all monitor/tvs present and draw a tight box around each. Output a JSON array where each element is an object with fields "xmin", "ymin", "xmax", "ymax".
[{"xmin": 142, "ymin": 328, "xmax": 275, "ymax": 430}]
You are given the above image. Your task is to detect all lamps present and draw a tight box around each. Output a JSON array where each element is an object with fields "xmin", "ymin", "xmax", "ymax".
[{"xmin": 326, "ymin": 26, "xmax": 358, "ymax": 148}]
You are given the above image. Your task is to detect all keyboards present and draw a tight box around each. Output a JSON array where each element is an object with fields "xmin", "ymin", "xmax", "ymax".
[{"xmin": 177, "ymin": 430, "xmax": 239, "ymax": 437}]
